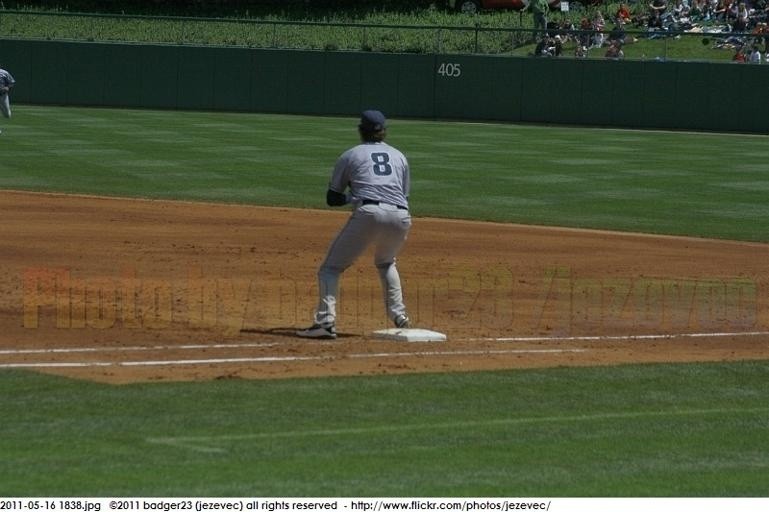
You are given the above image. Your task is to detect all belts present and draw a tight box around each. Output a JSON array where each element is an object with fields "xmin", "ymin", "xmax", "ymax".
[{"xmin": 362, "ymin": 199, "xmax": 407, "ymax": 210}]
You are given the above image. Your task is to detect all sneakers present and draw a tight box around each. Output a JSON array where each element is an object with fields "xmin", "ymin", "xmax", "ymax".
[
  {"xmin": 394, "ymin": 316, "xmax": 409, "ymax": 327},
  {"xmin": 295, "ymin": 325, "xmax": 338, "ymax": 339}
]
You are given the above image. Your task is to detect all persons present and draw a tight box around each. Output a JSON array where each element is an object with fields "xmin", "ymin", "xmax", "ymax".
[
  {"xmin": 294, "ymin": 108, "xmax": 411, "ymax": 339},
  {"xmin": 0, "ymin": 68, "xmax": 15, "ymax": 118},
  {"xmin": 532, "ymin": 0, "xmax": 768, "ymax": 61}
]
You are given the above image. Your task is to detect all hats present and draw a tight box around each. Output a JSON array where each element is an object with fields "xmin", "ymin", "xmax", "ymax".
[{"xmin": 359, "ymin": 109, "xmax": 386, "ymax": 131}]
